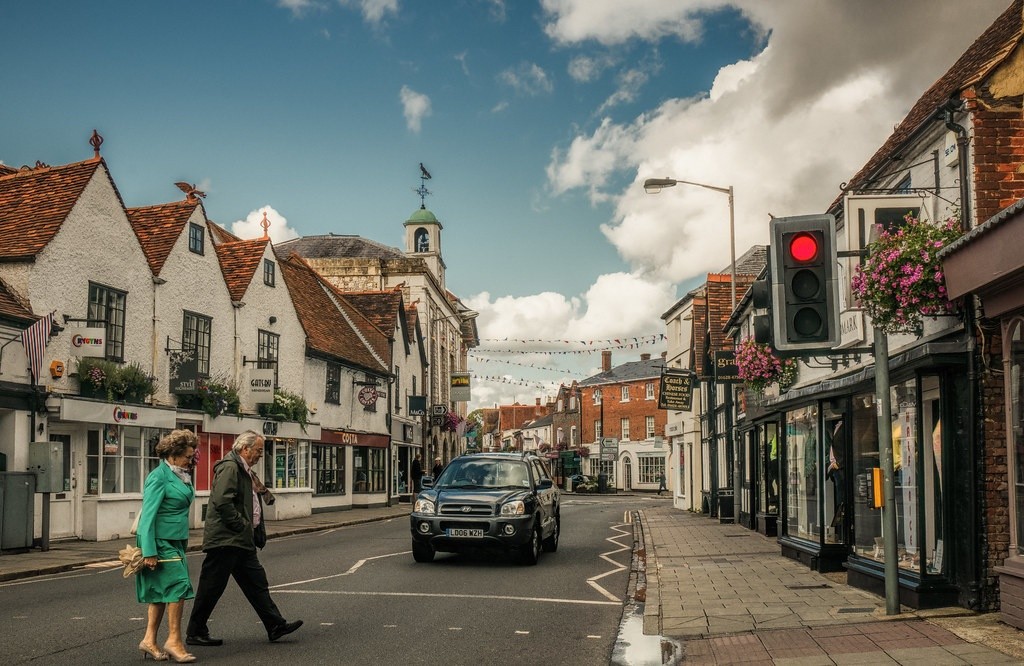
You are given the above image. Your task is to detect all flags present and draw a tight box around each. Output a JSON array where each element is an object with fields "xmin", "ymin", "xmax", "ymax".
[
  {"xmin": 534, "ymin": 434, "xmax": 540, "ymax": 446},
  {"xmin": 19, "ymin": 313, "xmax": 54, "ymax": 385}
]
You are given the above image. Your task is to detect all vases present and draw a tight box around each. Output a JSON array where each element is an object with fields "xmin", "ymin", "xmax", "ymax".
[
  {"xmin": 228, "ymin": 404, "xmax": 240, "ymax": 414},
  {"xmin": 119, "ymin": 391, "xmax": 146, "ymax": 403},
  {"xmin": 81, "ymin": 382, "xmax": 108, "ymax": 399},
  {"xmin": 183, "ymin": 395, "xmax": 205, "ymax": 409}
]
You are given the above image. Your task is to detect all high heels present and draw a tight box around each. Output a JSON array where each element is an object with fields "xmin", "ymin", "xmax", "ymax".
[
  {"xmin": 163, "ymin": 643, "xmax": 196, "ymax": 663},
  {"xmin": 138, "ymin": 641, "xmax": 169, "ymax": 662}
]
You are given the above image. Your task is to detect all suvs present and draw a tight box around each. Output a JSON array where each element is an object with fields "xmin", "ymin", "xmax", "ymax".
[{"xmin": 408, "ymin": 449, "xmax": 560, "ymax": 566}]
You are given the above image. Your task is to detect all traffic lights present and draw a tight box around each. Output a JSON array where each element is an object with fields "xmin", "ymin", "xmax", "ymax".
[{"xmin": 769, "ymin": 215, "xmax": 841, "ymax": 350}]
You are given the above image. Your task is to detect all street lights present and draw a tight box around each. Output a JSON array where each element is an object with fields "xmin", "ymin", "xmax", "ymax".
[{"xmin": 641, "ymin": 176, "xmax": 739, "ymax": 314}]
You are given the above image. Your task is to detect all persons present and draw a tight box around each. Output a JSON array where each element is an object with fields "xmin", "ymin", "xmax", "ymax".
[
  {"xmin": 657, "ymin": 471, "xmax": 668, "ymax": 495},
  {"xmin": 185, "ymin": 430, "xmax": 304, "ymax": 646},
  {"xmin": 433, "ymin": 456, "xmax": 444, "ymax": 481},
  {"xmin": 411, "ymin": 454, "xmax": 425, "ymax": 494},
  {"xmin": 135, "ymin": 430, "xmax": 196, "ymax": 663}
]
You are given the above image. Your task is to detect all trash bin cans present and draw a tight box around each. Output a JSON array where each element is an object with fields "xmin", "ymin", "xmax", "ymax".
[
  {"xmin": 717, "ymin": 487, "xmax": 734, "ymax": 524},
  {"xmin": 598, "ymin": 474, "xmax": 608, "ymax": 493}
]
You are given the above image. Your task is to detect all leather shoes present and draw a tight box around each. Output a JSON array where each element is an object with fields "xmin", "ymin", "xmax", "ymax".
[
  {"xmin": 186, "ymin": 634, "xmax": 223, "ymax": 645},
  {"xmin": 269, "ymin": 620, "xmax": 303, "ymax": 640}
]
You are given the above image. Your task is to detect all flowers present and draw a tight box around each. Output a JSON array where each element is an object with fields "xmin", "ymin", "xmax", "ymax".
[
  {"xmin": 442, "ymin": 412, "xmax": 460, "ymax": 431},
  {"xmin": 261, "ymin": 389, "xmax": 308, "ymax": 422},
  {"xmin": 77, "ymin": 355, "xmax": 119, "ymax": 386},
  {"xmin": 555, "ymin": 442, "xmax": 567, "ymax": 450},
  {"xmin": 848, "ymin": 210, "xmax": 972, "ymax": 335},
  {"xmin": 180, "ymin": 376, "xmax": 244, "ymax": 415},
  {"xmin": 577, "ymin": 447, "xmax": 589, "ymax": 457},
  {"xmin": 538, "ymin": 443, "xmax": 550, "ymax": 453},
  {"xmin": 735, "ymin": 338, "xmax": 796, "ymax": 393},
  {"xmin": 113, "ymin": 361, "xmax": 159, "ymax": 395}
]
